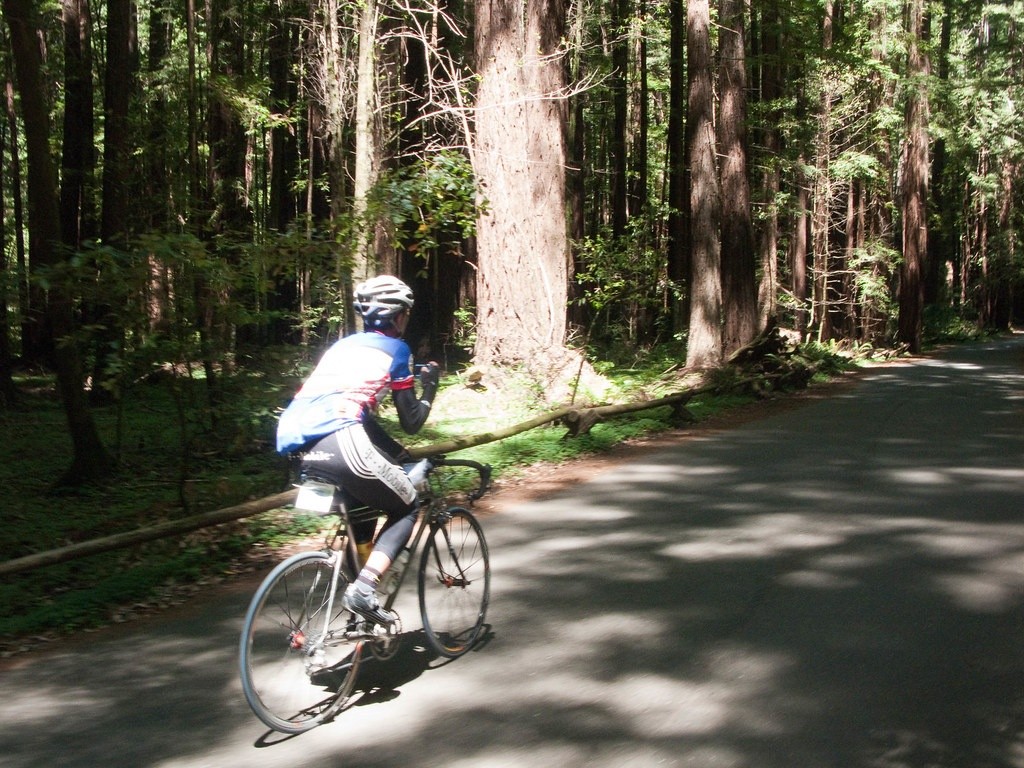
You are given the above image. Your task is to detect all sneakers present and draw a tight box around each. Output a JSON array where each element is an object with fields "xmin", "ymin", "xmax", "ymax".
[
  {"xmin": 341, "ymin": 583, "xmax": 395, "ymax": 626},
  {"xmin": 344, "ymin": 619, "xmax": 356, "ymax": 632}
]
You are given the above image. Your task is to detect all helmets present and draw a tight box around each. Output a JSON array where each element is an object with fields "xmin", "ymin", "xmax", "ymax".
[{"xmin": 353, "ymin": 275, "xmax": 414, "ymax": 319}]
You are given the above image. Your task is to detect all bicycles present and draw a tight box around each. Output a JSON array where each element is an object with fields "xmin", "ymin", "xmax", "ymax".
[{"xmin": 238, "ymin": 455, "xmax": 493, "ymax": 734}]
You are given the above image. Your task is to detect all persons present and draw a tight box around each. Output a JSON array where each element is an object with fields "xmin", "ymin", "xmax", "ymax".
[{"xmin": 276, "ymin": 274, "xmax": 439, "ymax": 641}]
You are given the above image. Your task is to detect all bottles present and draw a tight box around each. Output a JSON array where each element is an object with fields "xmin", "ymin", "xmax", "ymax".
[{"xmin": 376, "ymin": 547, "xmax": 410, "ymax": 596}]
